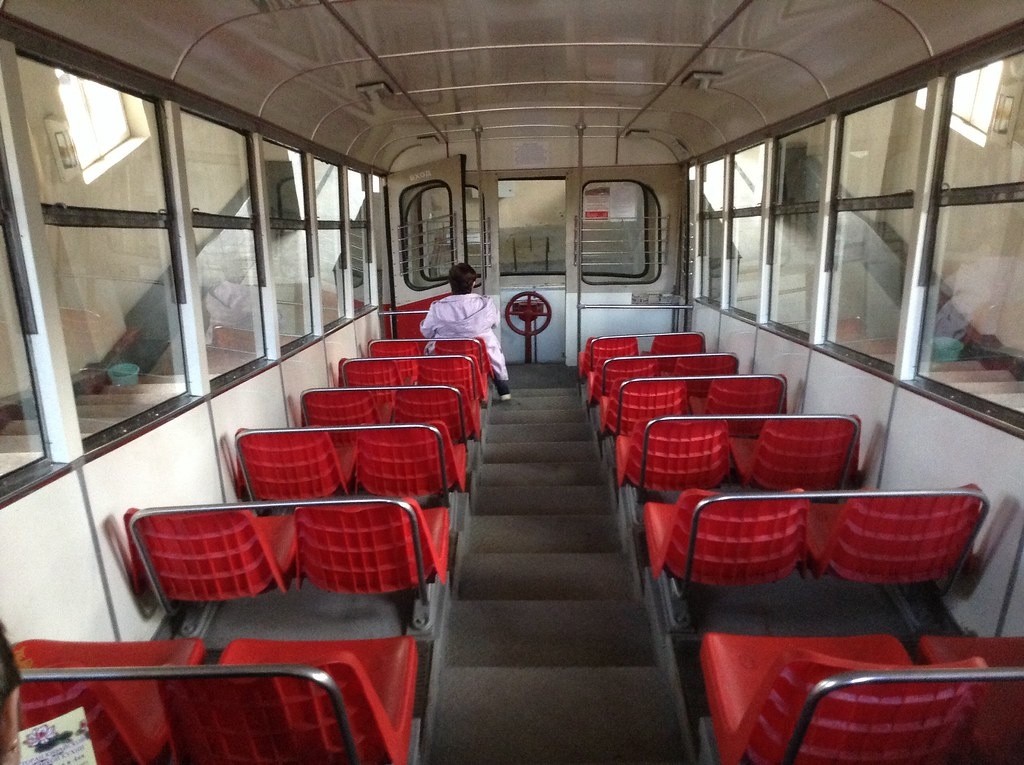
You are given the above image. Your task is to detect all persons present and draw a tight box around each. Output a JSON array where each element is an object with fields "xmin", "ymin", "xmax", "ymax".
[
  {"xmin": 0, "ymin": 620, "xmax": 22, "ymax": 765},
  {"xmin": 200, "ymin": 266, "xmax": 254, "ymax": 349},
  {"xmin": 420, "ymin": 263, "xmax": 510, "ymax": 401}
]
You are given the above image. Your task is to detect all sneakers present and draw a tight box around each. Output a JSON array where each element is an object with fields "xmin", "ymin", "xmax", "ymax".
[{"xmin": 498, "ymin": 386, "xmax": 511, "ymax": 400}]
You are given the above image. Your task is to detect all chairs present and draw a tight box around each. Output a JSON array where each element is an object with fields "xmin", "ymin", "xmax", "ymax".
[
  {"xmin": 576, "ymin": 330, "xmax": 1024, "ymax": 764},
  {"xmin": 15, "ymin": 334, "xmax": 487, "ymax": 765}
]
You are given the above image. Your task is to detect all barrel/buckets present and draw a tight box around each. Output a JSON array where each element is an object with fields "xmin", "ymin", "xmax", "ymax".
[
  {"xmin": 932, "ymin": 336, "xmax": 964, "ymax": 362},
  {"xmin": 108, "ymin": 363, "xmax": 140, "ymax": 385}
]
